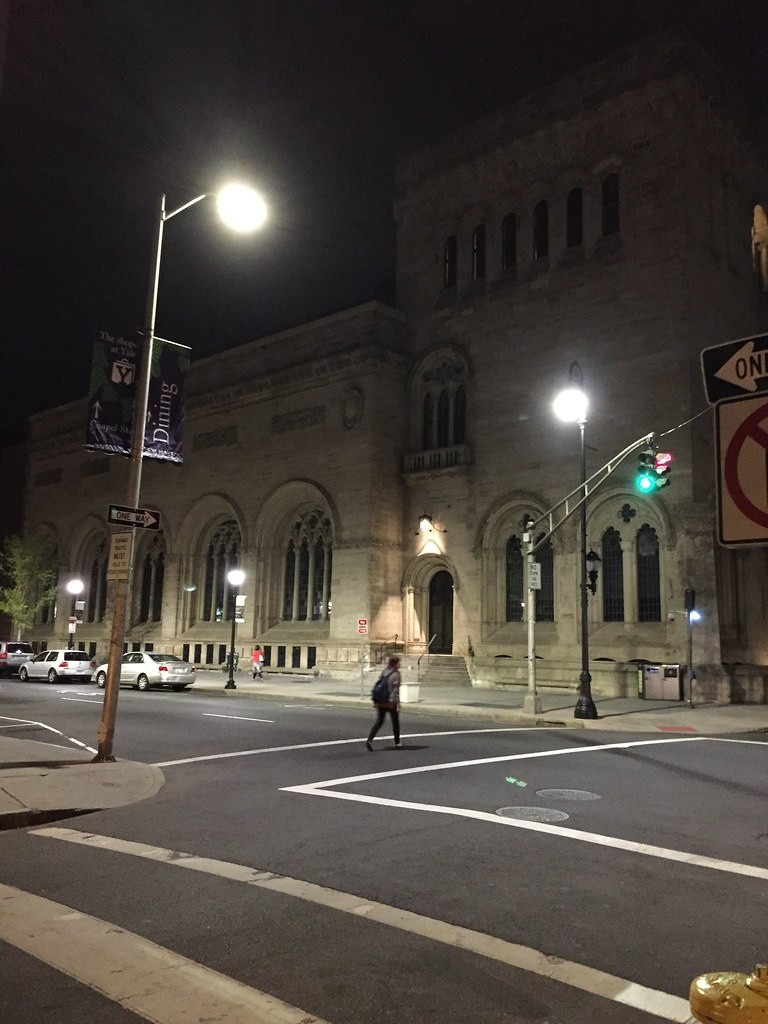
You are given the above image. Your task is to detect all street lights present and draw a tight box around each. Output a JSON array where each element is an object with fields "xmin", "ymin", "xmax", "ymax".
[
  {"xmin": 93, "ymin": 174, "xmax": 270, "ymax": 761},
  {"xmin": 65, "ymin": 577, "xmax": 86, "ymax": 645},
  {"xmin": 553, "ymin": 359, "xmax": 600, "ymax": 721},
  {"xmin": 225, "ymin": 569, "xmax": 246, "ymax": 689}
]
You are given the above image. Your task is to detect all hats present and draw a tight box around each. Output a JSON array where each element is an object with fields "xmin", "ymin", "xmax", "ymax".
[{"xmin": 389, "ymin": 656, "xmax": 400, "ymax": 666}]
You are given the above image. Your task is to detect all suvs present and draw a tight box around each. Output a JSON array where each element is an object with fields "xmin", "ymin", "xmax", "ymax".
[{"xmin": 0, "ymin": 641, "xmax": 35, "ymax": 678}]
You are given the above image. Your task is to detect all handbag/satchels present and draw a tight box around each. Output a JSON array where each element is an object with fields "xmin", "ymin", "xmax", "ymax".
[{"xmin": 258, "ymin": 654, "xmax": 265, "ymax": 662}]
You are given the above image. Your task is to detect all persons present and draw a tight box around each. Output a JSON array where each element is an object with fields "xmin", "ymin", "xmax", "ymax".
[
  {"xmin": 226, "ymin": 652, "xmax": 231, "ymax": 672},
  {"xmin": 364, "ymin": 657, "xmax": 404, "ymax": 752},
  {"xmin": 234, "ymin": 652, "xmax": 238, "ymax": 672},
  {"xmin": 249, "ymin": 645, "xmax": 264, "ymax": 681}
]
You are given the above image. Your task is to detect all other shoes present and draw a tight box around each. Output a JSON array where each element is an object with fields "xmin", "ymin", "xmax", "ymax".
[
  {"xmin": 365, "ymin": 741, "xmax": 373, "ymax": 752},
  {"xmin": 260, "ymin": 678, "xmax": 264, "ymax": 681},
  {"xmin": 251, "ymin": 677, "xmax": 255, "ymax": 681},
  {"xmin": 395, "ymin": 743, "xmax": 403, "ymax": 747}
]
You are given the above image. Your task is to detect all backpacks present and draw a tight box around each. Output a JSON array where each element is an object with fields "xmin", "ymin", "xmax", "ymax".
[{"xmin": 370, "ymin": 668, "xmax": 398, "ymax": 705}]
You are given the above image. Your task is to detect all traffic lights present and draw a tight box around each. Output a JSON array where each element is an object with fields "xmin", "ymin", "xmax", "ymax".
[
  {"xmin": 637, "ymin": 449, "xmax": 653, "ymax": 497},
  {"xmin": 653, "ymin": 452, "xmax": 674, "ymax": 496}
]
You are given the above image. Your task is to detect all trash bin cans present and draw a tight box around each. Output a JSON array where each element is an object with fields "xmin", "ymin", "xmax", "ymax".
[
  {"xmin": 660, "ymin": 664, "xmax": 681, "ymax": 701},
  {"xmin": 644, "ymin": 664, "xmax": 662, "ymax": 700}
]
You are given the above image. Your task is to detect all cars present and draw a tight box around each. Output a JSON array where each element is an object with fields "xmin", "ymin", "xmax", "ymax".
[
  {"xmin": 17, "ymin": 649, "xmax": 95, "ymax": 684},
  {"xmin": 92, "ymin": 651, "xmax": 197, "ymax": 690}
]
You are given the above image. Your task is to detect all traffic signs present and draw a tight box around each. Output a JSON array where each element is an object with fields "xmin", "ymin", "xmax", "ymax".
[{"xmin": 106, "ymin": 502, "xmax": 163, "ymax": 533}]
